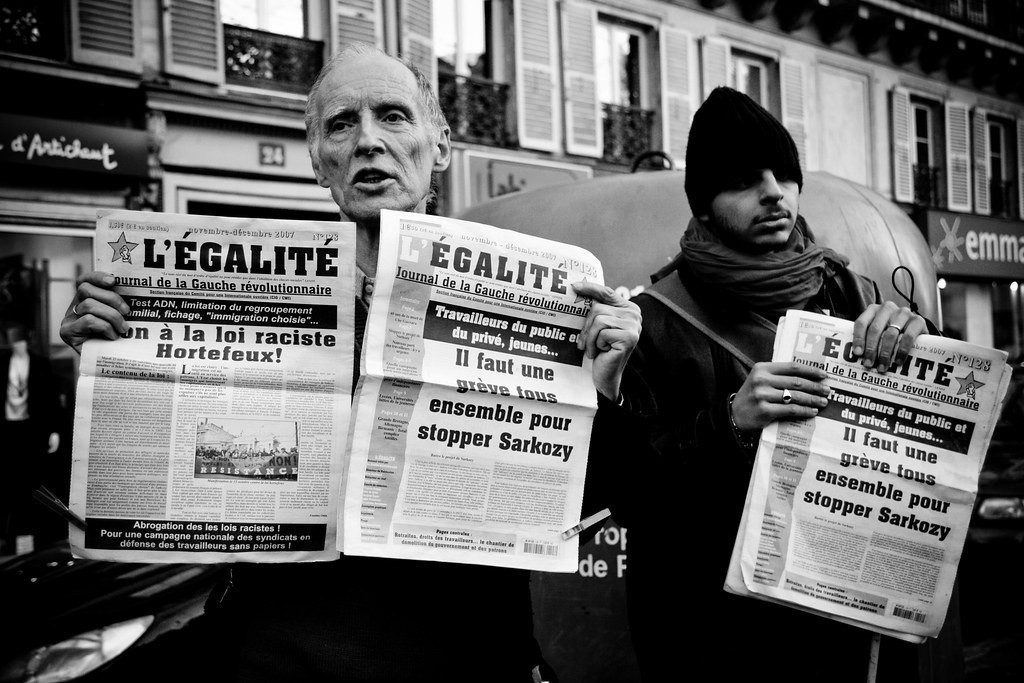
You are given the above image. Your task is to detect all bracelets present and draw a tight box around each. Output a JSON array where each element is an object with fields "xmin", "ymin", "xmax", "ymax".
[{"xmin": 728, "ymin": 400, "xmax": 757, "ymax": 449}]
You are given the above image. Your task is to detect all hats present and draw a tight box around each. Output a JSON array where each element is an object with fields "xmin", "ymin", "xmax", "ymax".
[{"xmin": 686, "ymin": 83, "xmax": 802, "ymax": 218}]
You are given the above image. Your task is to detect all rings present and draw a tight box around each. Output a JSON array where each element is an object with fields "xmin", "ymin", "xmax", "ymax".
[
  {"xmin": 784, "ymin": 389, "xmax": 791, "ymax": 403},
  {"xmin": 73, "ymin": 304, "xmax": 78, "ymax": 313},
  {"xmin": 888, "ymin": 324, "xmax": 901, "ymax": 333}
]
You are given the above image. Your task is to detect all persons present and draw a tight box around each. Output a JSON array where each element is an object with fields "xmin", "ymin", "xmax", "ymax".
[
  {"xmin": 59, "ymin": 44, "xmax": 642, "ymax": 683},
  {"xmin": 0, "ymin": 327, "xmax": 63, "ymax": 557},
  {"xmin": 615, "ymin": 86, "xmax": 942, "ymax": 683}
]
